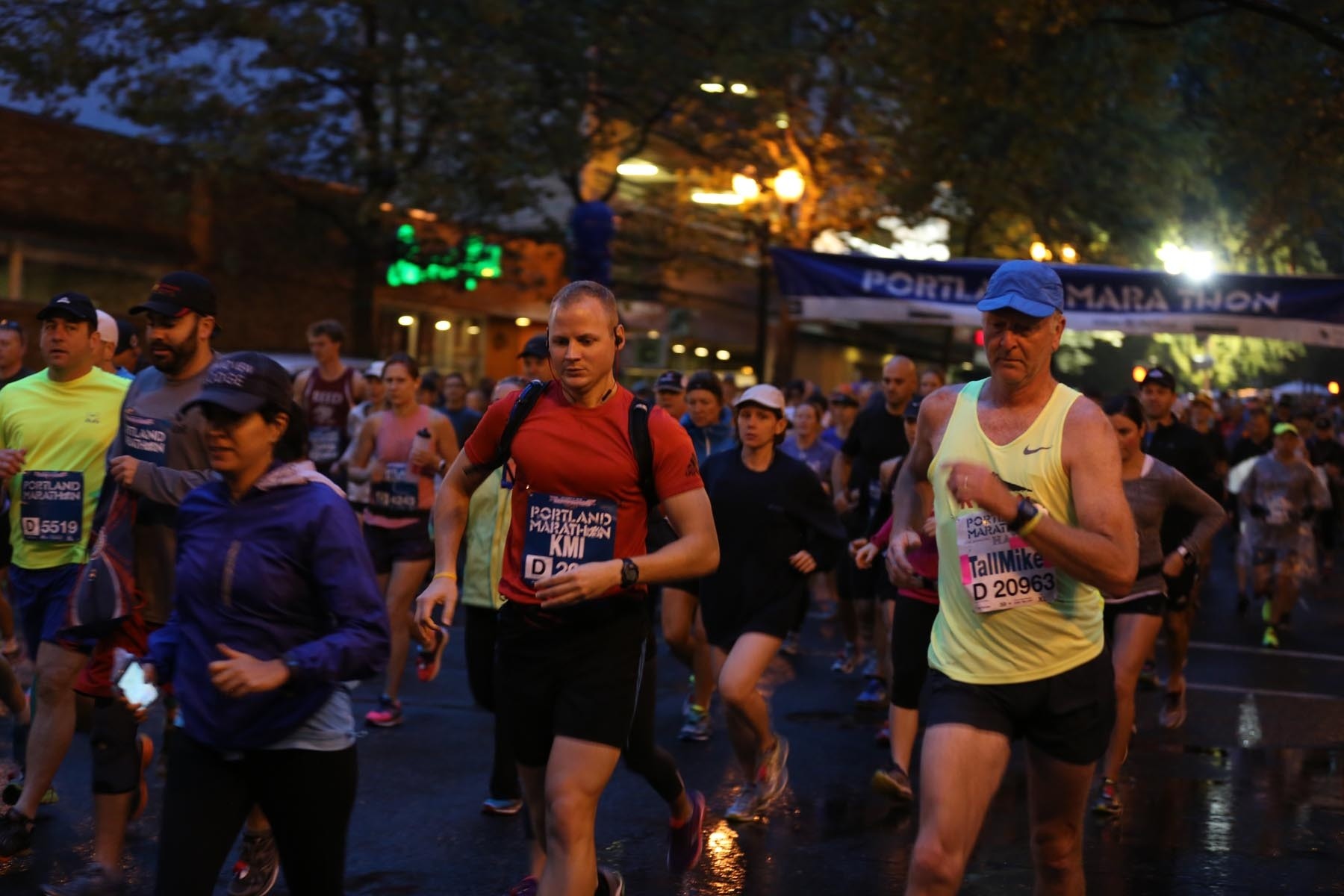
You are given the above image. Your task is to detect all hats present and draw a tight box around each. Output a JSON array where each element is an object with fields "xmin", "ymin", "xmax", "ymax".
[
  {"xmin": 95, "ymin": 309, "xmax": 119, "ymax": 347},
  {"xmin": 976, "ymin": 259, "xmax": 1064, "ymax": 317},
  {"xmin": 1274, "ymin": 422, "xmax": 1299, "ymax": 436},
  {"xmin": 128, "ymin": 270, "xmax": 216, "ymax": 317},
  {"xmin": 34, "ymin": 291, "xmax": 98, "ymax": 323},
  {"xmin": 653, "ymin": 371, "xmax": 683, "ymax": 394},
  {"xmin": 517, "ymin": 334, "xmax": 550, "ymax": 360},
  {"xmin": 686, "ymin": 371, "xmax": 724, "ymax": 398},
  {"xmin": 733, "ymin": 383, "xmax": 795, "ymax": 429},
  {"xmin": 1139, "ymin": 367, "xmax": 1178, "ymax": 392},
  {"xmin": 178, "ymin": 350, "xmax": 293, "ymax": 416}
]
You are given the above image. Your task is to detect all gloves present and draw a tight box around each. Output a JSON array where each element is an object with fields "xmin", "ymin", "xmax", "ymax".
[
  {"xmin": 1299, "ymin": 503, "xmax": 1316, "ymax": 522},
  {"xmin": 1248, "ymin": 503, "xmax": 1272, "ymax": 520}
]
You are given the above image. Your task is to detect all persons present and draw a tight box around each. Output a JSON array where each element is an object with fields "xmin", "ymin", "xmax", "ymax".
[
  {"xmin": 700, "ymin": 384, "xmax": 837, "ymax": 822},
  {"xmin": 1239, "ymin": 422, "xmax": 1333, "ymax": 649},
  {"xmin": 119, "ymin": 350, "xmax": 391, "ymax": 896},
  {"xmin": 1095, "ymin": 393, "xmax": 1227, "ymax": 814},
  {"xmin": 414, "ymin": 280, "xmax": 720, "ymax": 896},
  {"xmin": 0, "ymin": 260, "xmax": 1344, "ymax": 896}
]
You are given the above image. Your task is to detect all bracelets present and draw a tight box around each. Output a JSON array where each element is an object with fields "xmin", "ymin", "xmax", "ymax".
[
  {"xmin": 1010, "ymin": 499, "xmax": 1038, "ymax": 531},
  {"xmin": 433, "ymin": 573, "xmax": 458, "ymax": 580},
  {"xmin": 437, "ymin": 459, "xmax": 446, "ymax": 474},
  {"xmin": 1018, "ymin": 502, "xmax": 1048, "ymax": 537},
  {"xmin": 337, "ymin": 460, "xmax": 344, "ymax": 469},
  {"xmin": 1176, "ymin": 545, "xmax": 1191, "ymax": 560}
]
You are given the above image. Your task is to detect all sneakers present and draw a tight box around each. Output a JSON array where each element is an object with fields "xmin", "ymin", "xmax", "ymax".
[
  {"xmin": 677, "ymin": 681, "xmax": 724, "ymax": 740},
  {"xmin": 1262, "ymin": 601, "xmax": 1272, "ymax": 621},
  {"xmin": 509, "ymin": 875, "xmax": 540, "ymax": 896},
  {"xmin": 1090, "ymin": 776, "xmax": 1124, "ymax": 815},
  {"xmin": 667, "ymin": 791, "xmax": 705, "ymax": 875},
  {"xmin": 227, "ymin": 833, "xmax": 281, "ymax": 895},
  {"xmin": 2, "ymin": 775, "xmax": 60, "ymax": 806},
  {"xmin": 862, "ymin": 659, "xmax": 879, "ymax": 676},
  {"xmin": 724, "ymin": 765, "xmax": 788, "ymax": 821},
  {"xmin": 1136, "ymin": 660, "xmax": 1156, "ymax": 688},
  {"xmin": 596, "ymin": 868, "xmax": 625, "ymax": 895},
  {"xmin": 1262, "ymin": 627, "xmax": 1278, "ymax": 647},
  {"xmin": 365, "ymin": 694, "xmax": 403, "ymax": 727},
  {"xmin": 752, "ymin": 732, "xmax": 789, "ymax": 801},
  {"xmin": 871, "ymin": 762, "xmax": 915, "ymax": 803},
  {"xmin": 876, "ymin": 722, "xmax": 892, "ymax": 745},
  {"xmin": 126, "ymin": 732, "xmax": 154, "ymax": 823},
  {"xmin": 1, "ymin": 807, "xmax": 35, "ymax": 861},
  {"xmin": 830, "ymin": 651, "xmax": 867, "ymax": 674},
  {"xmin": 480, "ymin": 798, "xmax": 524, "ymax": 813},
  {"xmin": 856, "ymin": 677, "xmax": 886, "ymax": 707},
  {"xmin": 781, "ymin": 631, "xmax": 803, "ymax": 655},
  {"xmin": 416, "ymin": 627, "xmax": 449, "ymax": 683},
  {"xmin": 39, "ymin": 861, "xmax": 128, "ymax": 896},
  {"xmin": 1159, "ymin": 676, "xmax": 1187, "ymax": 731}
]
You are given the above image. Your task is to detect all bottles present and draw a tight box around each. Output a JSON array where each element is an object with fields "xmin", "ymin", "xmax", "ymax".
[{"xmin": 403, "ymin": 428, "xmax": 432, "ymax": 483}]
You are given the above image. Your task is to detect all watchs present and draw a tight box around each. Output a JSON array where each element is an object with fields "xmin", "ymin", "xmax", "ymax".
[{"xmin": 620, "ymin": 558, "xmax": 639, "ymax": 588}]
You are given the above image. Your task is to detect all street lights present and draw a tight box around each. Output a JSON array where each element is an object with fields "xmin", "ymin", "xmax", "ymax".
[{"xmin": 730, "ymin": 157, "xmax": 805, "ymax": 388}]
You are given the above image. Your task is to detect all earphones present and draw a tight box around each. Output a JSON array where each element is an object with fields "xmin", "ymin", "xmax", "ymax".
[{"xmin": 614, "ymin": 337, "xmax": 624, "ymax": 347}]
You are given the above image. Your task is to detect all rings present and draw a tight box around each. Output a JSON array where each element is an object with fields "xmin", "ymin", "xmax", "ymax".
[{"xmin": 964, "ymin": 476, "xmax": 968, "ymax": 489}]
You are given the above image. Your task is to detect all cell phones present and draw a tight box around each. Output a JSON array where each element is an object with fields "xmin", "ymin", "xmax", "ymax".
[{"xmin": 116, "ymin": 659, "xmax": 158, "ymax": 713}]
